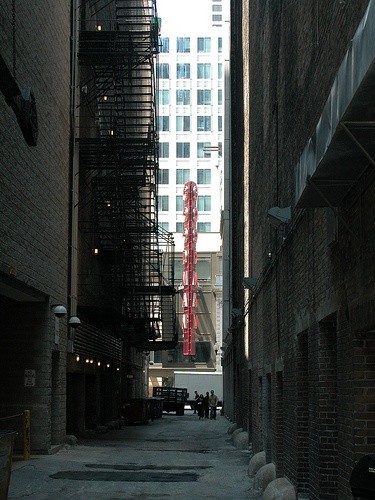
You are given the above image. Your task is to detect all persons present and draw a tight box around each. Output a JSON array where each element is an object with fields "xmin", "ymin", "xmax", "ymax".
[
  {"xmin": 194, "ymin": 390, "xmax": 210, "ymax": 419},
  {"xmin": 209, "ymin": 390, "xmax": 219, "ymax": 420}
]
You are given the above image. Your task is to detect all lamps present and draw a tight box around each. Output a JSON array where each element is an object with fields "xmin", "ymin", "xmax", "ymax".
[
  {"xmin": 240, "ymin": 278, "xmax": 257, "ymax": 290},
  {"xmin": 50, "ymin": 303, "xmax": 80, "ymax": 328},
  {"xmin": 232, "ymin": 308, "xmax": 242, "ymax": 318},
  {"xmin": 266, "ymin": 205, "xmax": 291, "ymax": 227}
]
